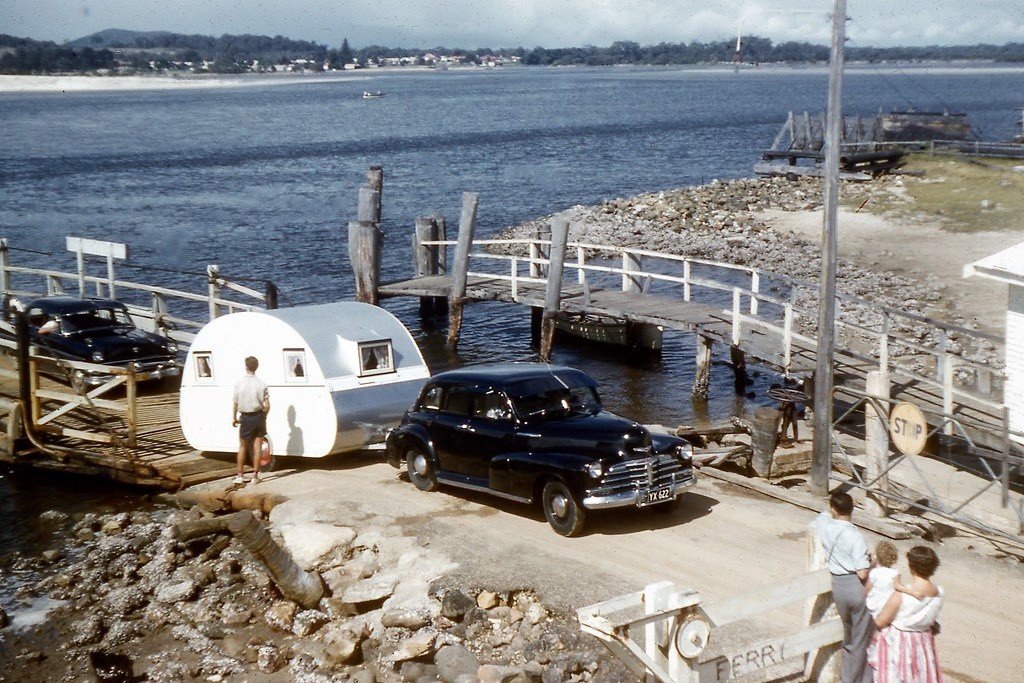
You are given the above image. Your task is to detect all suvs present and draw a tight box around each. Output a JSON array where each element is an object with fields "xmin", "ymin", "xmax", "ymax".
[{"xmin": 385, "ymin": 360, "xmax": 697, "ymax": 538}]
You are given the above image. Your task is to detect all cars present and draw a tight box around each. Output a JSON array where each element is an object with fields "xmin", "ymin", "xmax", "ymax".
[{"xmin": 0, "ymin": 295, "xmax": 180, "ymax": 396}]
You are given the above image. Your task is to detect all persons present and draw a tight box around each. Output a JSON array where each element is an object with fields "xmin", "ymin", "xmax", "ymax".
[
  {"xmin": 232, "ymin": 357, "xmax": 270, "ymax": 484},
  {"xmin": 818, "ymin": 493, "xmax": 943, "ymax": 683},
  {"xmin": 486, "ymin": 395, "xmax": 514, "ymax": 418},
  {"xmin": 38, "ymin": 314, "xmax": 61, "ymax": 334}
]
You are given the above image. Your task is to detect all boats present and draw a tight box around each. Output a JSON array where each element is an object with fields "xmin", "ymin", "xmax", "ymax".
[{"xmin": 363, "ymin": 94, "xmax": 386, "ymax": 99}]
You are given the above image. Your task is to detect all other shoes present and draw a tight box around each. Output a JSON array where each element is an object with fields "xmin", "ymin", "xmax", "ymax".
[
  {"xmin": 251, "ymin": 478, "xmax": 262, "ymax": 485},
  {"xmin": 232, "ymin": 476, "xmax": 243, "ymax": 484}
]
